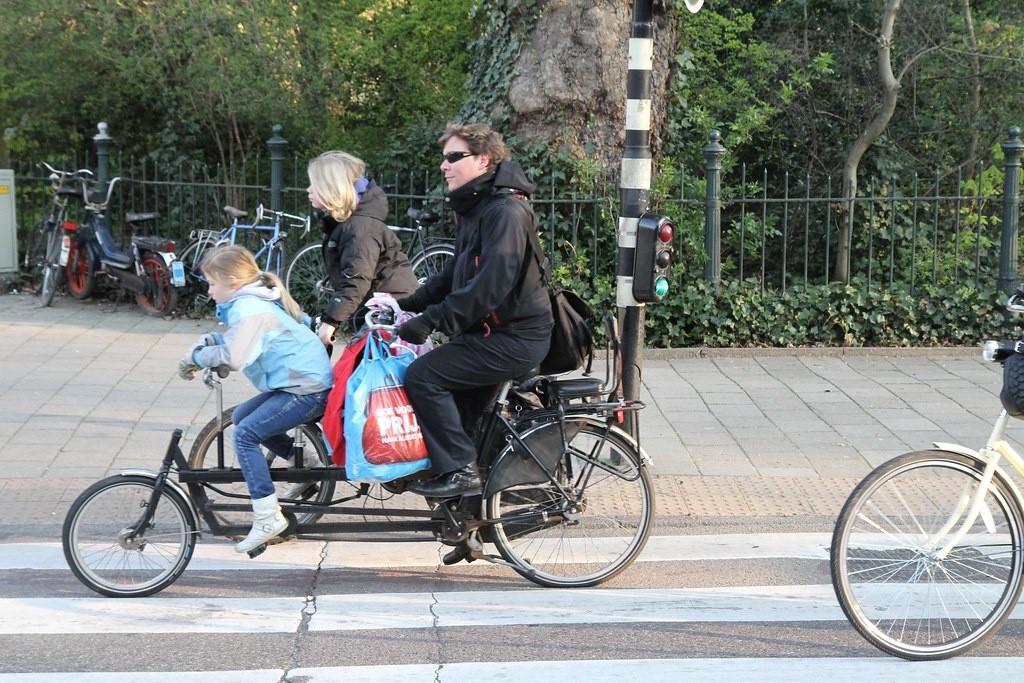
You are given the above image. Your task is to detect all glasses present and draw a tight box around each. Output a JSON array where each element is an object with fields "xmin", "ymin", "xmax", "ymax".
[{"xmin": 443, "ymin": 150, "xmax": 476, "ymax": 162}]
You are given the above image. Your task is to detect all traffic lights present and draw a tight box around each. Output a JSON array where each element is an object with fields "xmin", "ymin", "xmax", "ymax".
[{"xmin": 633, "ymin": 213, "xmax": 674, "ymax": 307}]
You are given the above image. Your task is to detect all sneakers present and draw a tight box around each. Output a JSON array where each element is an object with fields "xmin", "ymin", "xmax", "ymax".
[
  {"xmin": 235, "ymin": 511, "xmax": 289, "ymax": 551},
  {"xmin": 284, "ymin": 460, "xmax": 325, "ymax": 501}
]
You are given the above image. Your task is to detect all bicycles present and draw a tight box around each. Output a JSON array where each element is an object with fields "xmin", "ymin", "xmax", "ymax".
[
  {"xmin": 285, "ymin": 207, "xmax": 458, "ymax": 320},
  {"xmin": 62, "ymin": 176, "xmax": 184, "ymax": 317},
  {"xmin": 187, "ymin": 333, "xmax": 337, "ymax": 542},
  {"xmin": 173, "ymin": 201, "xmax": 312, "ymax": 311},
  {"xmin": 828, "ymin": 338, "xmax": 1024, "ymax": 662},
  {"xmin": 21, "ymin": 160, "xmax": 94, "ymax": 308},
  {"xmin": 62, "ymin": 364, "xmax": 658, "ymax": 588}
]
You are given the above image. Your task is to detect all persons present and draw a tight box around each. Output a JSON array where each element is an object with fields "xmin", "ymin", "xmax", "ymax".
[
  {"xmin": 395, "ymin": 123, "xmax": 554, "ymax": 565},
  {"xmin": 178, "ymin": 244, "xmax": 332, "ymax": 554},
  {"xmin": 306, "ymin": 150, "xmax": 420, "ymax": 345}
]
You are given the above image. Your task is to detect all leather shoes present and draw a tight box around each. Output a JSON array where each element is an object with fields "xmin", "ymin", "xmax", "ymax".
[
  {"xmin": 444, "ymin": 543, "xmax": 468, "ymax": 565},
  {"xmin": 411, "ymin": 460, "xmax": 482, "ymax": 497}
]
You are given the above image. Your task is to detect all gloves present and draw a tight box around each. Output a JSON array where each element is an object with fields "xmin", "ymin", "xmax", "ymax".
[
  {"xmin": 179, "ymin": 353, "xmax": 198, "ymax": 380},
  {"xmin": 198, "ymin": 333, "xmax": 214, "ymax": 346},
  {"xmin": 398, "ymin": 317, "xmax": 431, "ymax": 345}
]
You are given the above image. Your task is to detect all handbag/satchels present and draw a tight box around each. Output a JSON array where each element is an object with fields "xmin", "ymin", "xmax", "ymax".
[
  {"xmin": 539, "ymin": 286, "xmax": 598, "ymax": 373},
  {"xmin": 323, "ymin": 313, "xmax": 430, "ymax": 482}
]
